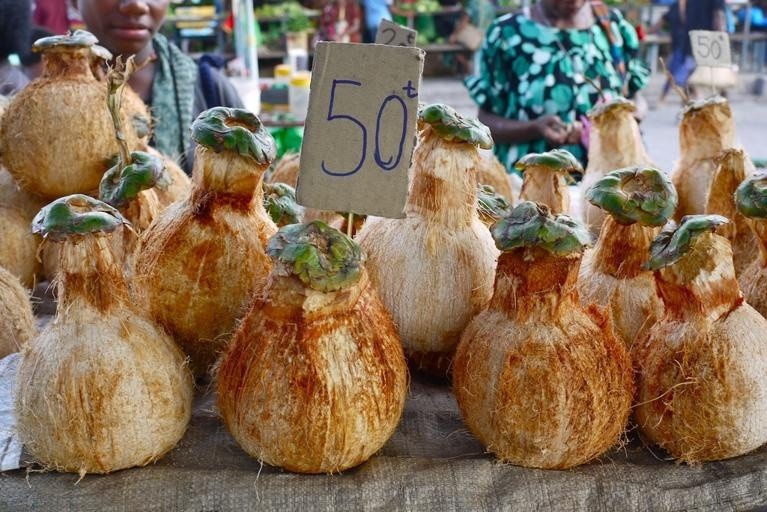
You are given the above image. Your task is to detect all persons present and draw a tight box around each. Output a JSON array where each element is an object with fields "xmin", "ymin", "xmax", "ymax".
[
  {"xmin": 1, "ymin": 0, "xmax": 88, "ymax": 101},
  {"xmin": 430, "ymin": 1, "xmax": 498, "ymax": 75},
  {"xmin": 663, "ymin": 0, "xmax": 767, "ymax": 106},
  {"xmin": 78, "ymin": 0, "xmax": 246, "ymax": 175},
  {"xmin": 465, "ymin": 0, "xmax": 652, "ymax": 185},
  {"xmin": 362, "ymin": 0, "xmax": 414, "ymax": 46},
  {"xmin": 312, "ymin": 1, "xmax": 363, "ymax": 43}
]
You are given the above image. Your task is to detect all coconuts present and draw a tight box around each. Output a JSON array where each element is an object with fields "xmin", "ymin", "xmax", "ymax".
[{"xmin": 0, "ymin": 28, "xmax": 767, "ymax": 475}]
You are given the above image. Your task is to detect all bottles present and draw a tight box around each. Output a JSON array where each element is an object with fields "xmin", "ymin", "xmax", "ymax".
[{"xmin": 259, "ymin": 64, "xmax": 311, "ymax": 119}]
